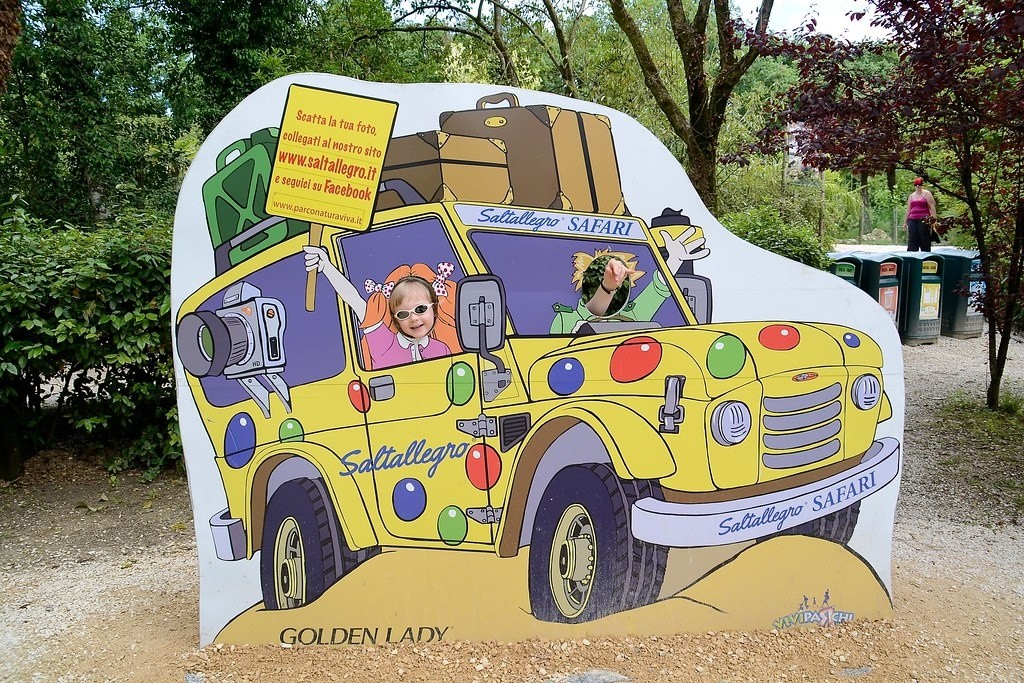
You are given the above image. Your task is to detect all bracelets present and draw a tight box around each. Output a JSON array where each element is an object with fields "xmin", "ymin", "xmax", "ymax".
[{"xmin": 601, "ymin": 278, "xmax": 618, "ymax": 295}]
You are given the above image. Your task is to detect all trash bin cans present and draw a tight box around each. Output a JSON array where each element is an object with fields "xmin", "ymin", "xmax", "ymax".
[
  {"xmin": 824, "ymin": 251, "xmax": 863, "ymax": 288},
  {"xmin": 935, "ymin": 247, "xmax": 986, "ymax": 341},
  {"xmin": 847, "ymin": 249, "xmax": 904, "ymax": 336},
  {"xmin": 893, "ymin": 251, "xmax": 945, "ymax": 347}
]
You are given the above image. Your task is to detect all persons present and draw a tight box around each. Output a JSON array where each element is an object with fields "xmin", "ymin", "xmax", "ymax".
[
  {"xmin": 903, "ymin": 178, "xmax": 936, "ymax": 252},
  {"xmin": 586, "ymin": 258, "xmax": 635, "ymax": 316},
  {"xmin": 390, "ymin": 277, "xmax": 438, "ymax": 339}
]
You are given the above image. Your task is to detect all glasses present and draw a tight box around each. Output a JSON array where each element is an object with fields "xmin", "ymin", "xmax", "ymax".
[
  {"xmin": 914, "ymin": 183, "xmax": 922, "ymax": 186},
  {"xmin": 392, "ymin": 303, "xmax": 436, "ymax": 321}
]
